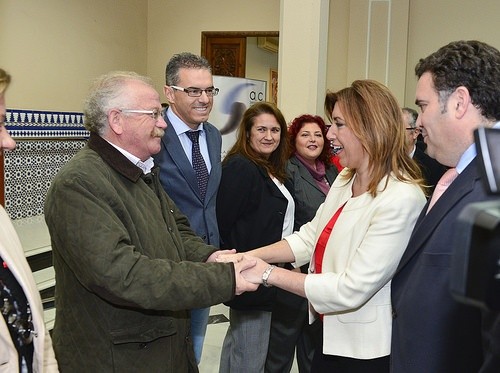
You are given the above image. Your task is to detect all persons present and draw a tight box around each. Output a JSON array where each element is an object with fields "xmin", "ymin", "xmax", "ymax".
[
  {"xmin": 43, "ymin": 70, "xmax": 258, "ymax": 373},
  {"xmin": 213, "ymin": 41, "xmax": 500, "ymax": 373},
  {"xmin": 0, "ymin": 69, "xmax": 59, "ymax": 373},
  {"xmin": 148, "ymin": 53, "xmax": 222, "ymax": 366}
]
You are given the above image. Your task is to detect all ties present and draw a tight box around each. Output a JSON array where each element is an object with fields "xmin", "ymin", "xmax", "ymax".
[{"xmin": 425, "ymin": 168, "xmax": 459, "ymax": 215}]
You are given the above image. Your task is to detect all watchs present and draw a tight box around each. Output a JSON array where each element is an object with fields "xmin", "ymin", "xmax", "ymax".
[{"xmin": 261, "ymin": 265, "xmax": 278, "ymax": 288}]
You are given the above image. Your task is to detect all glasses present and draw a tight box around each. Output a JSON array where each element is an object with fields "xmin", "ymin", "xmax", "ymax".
[
  {"xmin": 170, "ymin": 86, "xmax": 219, "ymax": 97},
  {"xmin": 119, "ymin": 109, "xmax": 164, "ymax": 120}
]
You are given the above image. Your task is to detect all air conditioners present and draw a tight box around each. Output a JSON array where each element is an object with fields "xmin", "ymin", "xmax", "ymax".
[{"xmin": 257, "ymin": 36, "xmax": 279, "ymax": 54}]
